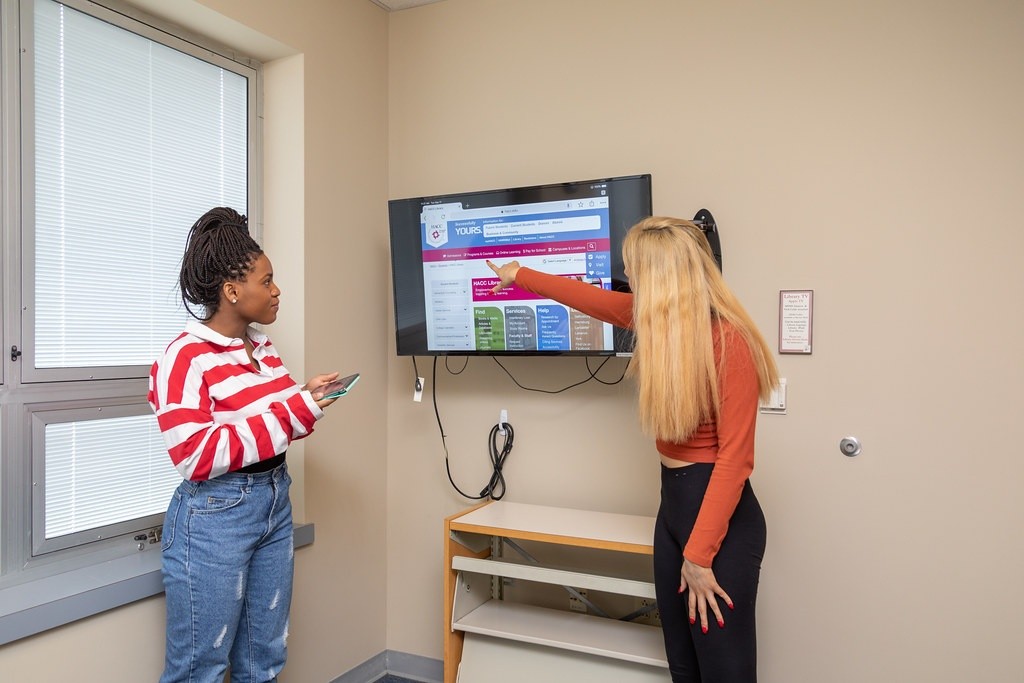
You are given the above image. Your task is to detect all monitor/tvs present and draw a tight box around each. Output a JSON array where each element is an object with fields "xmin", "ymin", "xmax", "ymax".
[{"xmin": 387, "ymin": 173, "xmax": 647, "ymax": 357}]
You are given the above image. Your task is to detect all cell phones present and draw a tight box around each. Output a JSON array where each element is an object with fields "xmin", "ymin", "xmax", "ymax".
[{"xmin": 310, "ymin": 373, "xmax": 361, "ymax": 402}]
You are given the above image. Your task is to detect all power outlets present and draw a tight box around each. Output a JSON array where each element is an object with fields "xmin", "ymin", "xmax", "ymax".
[
  {"xmin": 640, "ymin": 598, "xmax": 661, "ymax": 622},
  {"xmin": 570, "ymin": 588, "xmax": 587, "ymax": 611}
]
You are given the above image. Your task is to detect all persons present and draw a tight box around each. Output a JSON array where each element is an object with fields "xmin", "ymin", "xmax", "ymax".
[
  {"xmin": 486, "ymin": 217, "xmax": 778, "ymax": 683},
  {"xmin": 148, "ymin": 207, "xmax": 341, "ymax": 683}
]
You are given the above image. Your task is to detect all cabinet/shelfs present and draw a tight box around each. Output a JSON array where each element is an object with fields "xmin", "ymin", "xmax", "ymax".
[{"xmin": 443, "ymin": 500, "xmax": 674, "ymax": 683}]
